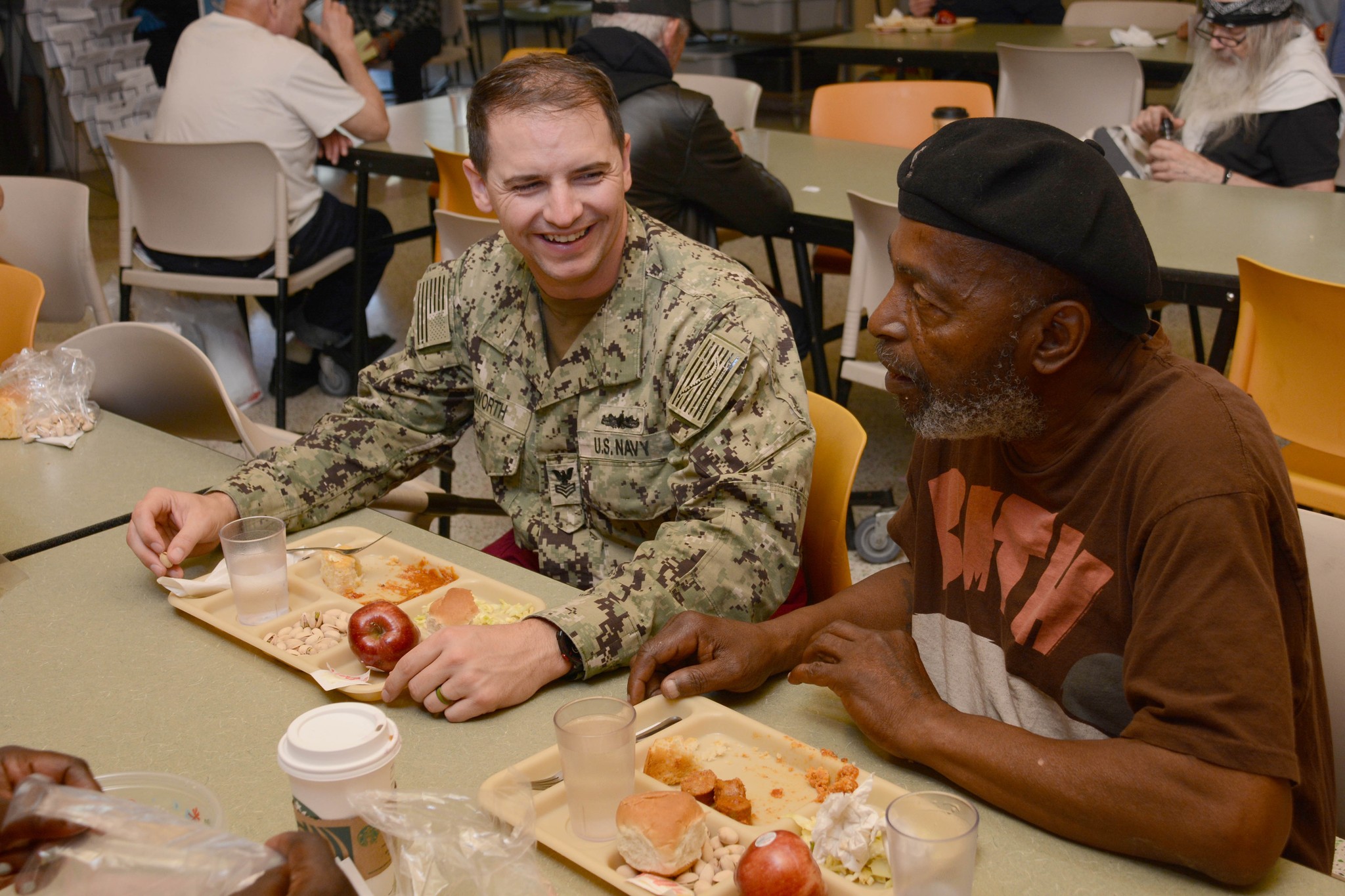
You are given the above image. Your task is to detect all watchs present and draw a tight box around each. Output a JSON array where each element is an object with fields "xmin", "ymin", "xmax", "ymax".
[{"xmin": 556, "ymin": 629, "xmax": 584, "ymax": 681}]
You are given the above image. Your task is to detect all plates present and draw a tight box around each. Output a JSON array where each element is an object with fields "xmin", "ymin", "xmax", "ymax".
[
  {"xmin": 479, "ymin": 689, "xmax": 975, "ymax": 896},
  {"xmin": 167, "ymin": 527, "xmax": 548, "ymax": 700},
  {"xmin": 865, "ymin": 15, "xmax": 977, "ymax": 33}
]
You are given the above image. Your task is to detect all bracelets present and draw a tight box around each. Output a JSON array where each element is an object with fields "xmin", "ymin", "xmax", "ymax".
[{"xmin": 1222, "ymin": 165, "xmax": 1233, "ymax": 184}]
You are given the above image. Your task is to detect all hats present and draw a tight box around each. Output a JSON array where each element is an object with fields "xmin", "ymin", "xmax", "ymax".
[
  {"xmin": 894, "ymin": 118, "xmax": 1164, "ymax": 304},
  {"xmin": 591, "ymin": 0, "xmax": 721, "ymax": 45}
]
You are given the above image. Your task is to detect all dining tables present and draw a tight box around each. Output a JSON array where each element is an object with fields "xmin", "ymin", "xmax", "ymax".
[
  {"xmin": 0, "ymin": 507, "xmax": 1344, "ymax": 895},
  {"xmin": 0, "ymin": 372, "xmax": 255, "ymax": 566}
]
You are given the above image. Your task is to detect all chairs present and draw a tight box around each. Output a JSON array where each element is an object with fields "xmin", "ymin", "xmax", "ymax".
[
  {"xmin": 803, "ymin": 80, "xmax": 997, "ymax": 389},
  {"xmin": 831, "ymin": 191, "xmax": 905, "ymax": 409},
  {"xmin": 806, "ymin": 385, "xmax": 870, "ymax": 606},
  {"xmin": 33, "ymin": 323, "xmax": 263, "ymax": 460},
  {"xmin": 99, "ymin": 130, "xmax": 367, "ymax": 434}
]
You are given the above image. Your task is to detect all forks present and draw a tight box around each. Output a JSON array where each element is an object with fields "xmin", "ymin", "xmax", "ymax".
[{"xmin": 287, "ymin": 530, "xmax": 392, "ymax": 556}]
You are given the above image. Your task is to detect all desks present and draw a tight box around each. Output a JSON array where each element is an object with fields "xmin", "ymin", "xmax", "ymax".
[
  {"xmin": 795, "ymin": 20, "xmax": 1193, "ymax": 112},
  {"xmin": 318, "ymin": 86, "xmax": 1343, "ymax": 378}
]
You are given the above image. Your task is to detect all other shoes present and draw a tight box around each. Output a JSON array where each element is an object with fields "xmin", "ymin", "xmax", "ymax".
[
  {"xmin": 267, "ymin": 347, "xmax": 320, "ymax": 397},
  {"xmin": 327, "ymin": 332, "xmax": 397, "ymax": 377}
]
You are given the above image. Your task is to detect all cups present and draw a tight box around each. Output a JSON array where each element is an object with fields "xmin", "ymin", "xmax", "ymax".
[
  {"xmin": 303, "ymin": 0, "xmax": 323, "ymax": 26},
  {"xmin": 277, "ymin": 699, "xmax": 404, "ymax": 895},
  {"xmin": 447, "ymin": 86, "xmax": 472, "ymax": 125},
  {"xmin": 217, "ymin": 516, "xmax": 291, "ymax": 627},
  {"xmin": 555, "ymin": 696, "xmax": 639, "ymax": 842},
  {"xmin": 886, "ymin": 790, "xmax": 978, "ymax": 896}
]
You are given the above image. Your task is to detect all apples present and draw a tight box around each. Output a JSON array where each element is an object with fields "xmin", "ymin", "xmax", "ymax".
[
  {"xmin": 347, "ymin": 601, "xmax": 422, "ymax": 673},
  {"xmin": 733, "ymin": 829, "xmax": 824, "ymax": 896}
]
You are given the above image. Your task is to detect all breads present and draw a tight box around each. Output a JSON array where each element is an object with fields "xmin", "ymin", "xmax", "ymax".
[
  {"xmin": 0, "ymin": 381, "xmax": 32, "ymax": 441},
  {"xmin": 426, "ymin": 587, "xmax": 480, "ymax": 634},
  {"xmin": 320, "ymin": 544, "xmax": 364, "ymax": 595},
  {"xmin": 615, "ymin": 790, "xmax": 710, "ymax": 877}
]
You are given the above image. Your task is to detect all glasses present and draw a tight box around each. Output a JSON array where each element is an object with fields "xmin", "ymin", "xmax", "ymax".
[{"xmin": 1195, "ymin": 16, "xmax": 1259, "ymax": 49}]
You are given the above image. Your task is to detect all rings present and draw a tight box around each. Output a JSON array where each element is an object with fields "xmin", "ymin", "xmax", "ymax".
[{"xmin": 436, "ymin": 687, "xmax": 457, "ymax": 707}]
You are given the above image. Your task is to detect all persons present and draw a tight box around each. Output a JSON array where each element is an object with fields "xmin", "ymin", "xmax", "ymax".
[
  {"xmin": 906, "ymin": 0, "xmax": 1066, "ymax": 26},
  {"xmin": 627, "ymin": 114, "xmax": 1345, "ymax": 896},
  {"xmin": 134, "ymin": 0, "xmax": 395, "ymax": 399},
  {"xmin": 560, "ymin": 2, "xmax": 813, "ymax": 367},
  {"xmin": 1127, "ymin": 0, "xmax": 1345, "ymax": 192},
  {"xmin": 0, "ymin": 745, "xmax": 354, "ymax": 896},
  {"xmin": 122, "ymin": 57, "xmax": 811, "ymax": 726},
  {"xmin": 321, "ymin": 1, "xmax": 444, "ymax": 103}
]
